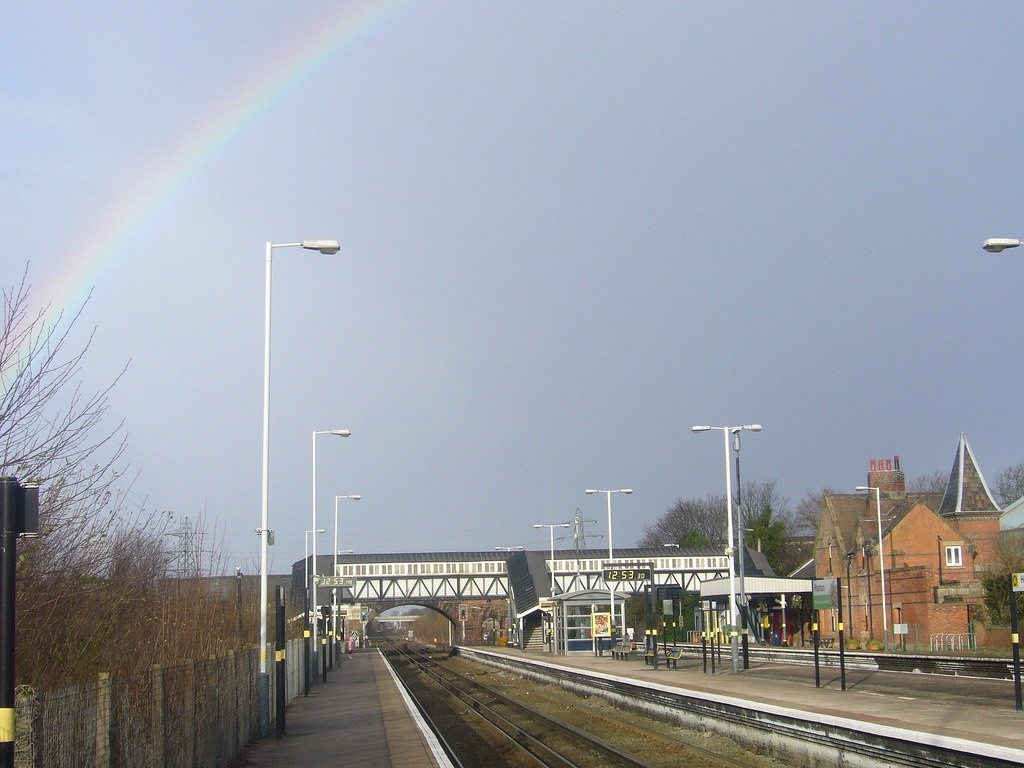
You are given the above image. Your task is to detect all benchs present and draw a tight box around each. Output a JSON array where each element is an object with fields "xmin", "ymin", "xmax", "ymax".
[
  {"xmin": 611, "ymin": 642, "xmax": 633, "ymax": 661},
  {"xmin": 805, "ymin": 634, "xmax": 834, "ymax": 648},
  {"xmin": 637, "ymin": 647, "xmax": 660, "ymax": 665},
  {"xmin": 666, "ymin": 647, "xmax": 683, "ymax": 669}
]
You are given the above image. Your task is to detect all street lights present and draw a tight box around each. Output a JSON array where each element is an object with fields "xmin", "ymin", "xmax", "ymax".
[
  {"xmin": 305, "ymin": 528, "xmax": 325, "ymax": 590},
  {"xmin": 256, "ymin": 238, "xmax": 344, "ymax": 676},
  {"xmin": 690, "ymin": 424, "xmax": 764, "ymax": 673},
  {"xmin": 856, "ymin": 485, "xmax": 889, "ymax": 652},
  {"xmin": 310, "ymin": 430, "xmax": 352, "ymax": 653},
  {"xmin": 534, "ymin": 524, "xmax": 571, "ymax": 654},
  {"xmin": 585, "ymin": 488, "xmax": 634, "ymax": 659},
  {"xmin": 332, "ymin": 494, "xmax": 362, "ymax": 666}
]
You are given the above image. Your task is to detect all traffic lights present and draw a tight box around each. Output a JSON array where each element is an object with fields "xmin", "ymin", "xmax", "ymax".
[
  {"xmin": 459, "ymin": 604, "xmax": 468, "ymax": 622},
  {"xmin": 360, "ymin": 607, "xmax": 367, "ymax": 623}
]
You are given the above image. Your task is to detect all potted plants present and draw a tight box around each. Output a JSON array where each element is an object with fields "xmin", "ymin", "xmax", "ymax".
[
  {"xmin": 868, "ymin": 641, "xmax": 881, "ymax": 650},
  {"xmin": 847, "ymin": 640, "xmax": 860, "ymax": 649}
]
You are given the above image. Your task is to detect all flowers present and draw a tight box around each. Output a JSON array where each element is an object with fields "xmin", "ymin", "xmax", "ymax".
[{"xmin": 790, "ymin": 595, "xmax": 803, "ymax": 609}]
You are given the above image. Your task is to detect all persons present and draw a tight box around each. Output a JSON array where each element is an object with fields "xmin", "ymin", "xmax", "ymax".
[{"xmin": 349, "ymin": 632, "xmax": 357, "ymax": 659}]
